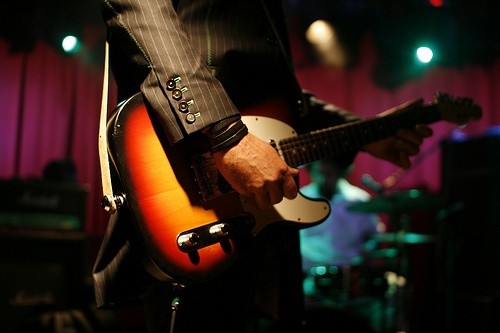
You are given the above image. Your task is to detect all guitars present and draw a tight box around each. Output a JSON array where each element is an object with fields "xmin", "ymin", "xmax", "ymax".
[{"xmin": 106, "ymin": 88, "xmax": 483, "ymax": 283}]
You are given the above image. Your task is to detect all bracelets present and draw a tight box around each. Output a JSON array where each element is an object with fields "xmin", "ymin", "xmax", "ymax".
[{"xmin": 209, "ymin": 121, "xmax": 248, "ymax": 153}]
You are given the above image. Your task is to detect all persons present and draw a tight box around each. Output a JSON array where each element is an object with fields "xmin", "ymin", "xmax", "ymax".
[
  {"xmin": 296, "ymin": 150, "xmax": 379, "ymax": 281},
  {"xmin": 98, "ymin": 0, "xmax": 435, "ymax": 333}
]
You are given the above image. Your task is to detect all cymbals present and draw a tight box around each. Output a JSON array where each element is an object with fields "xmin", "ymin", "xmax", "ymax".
[
  {"xmin": 346, "ymin": 190, "xmax": 450, "ymax": 213},
  {"xmin": 359, "ymin": 230, "xmax": 437, "ymax": 245}
]
